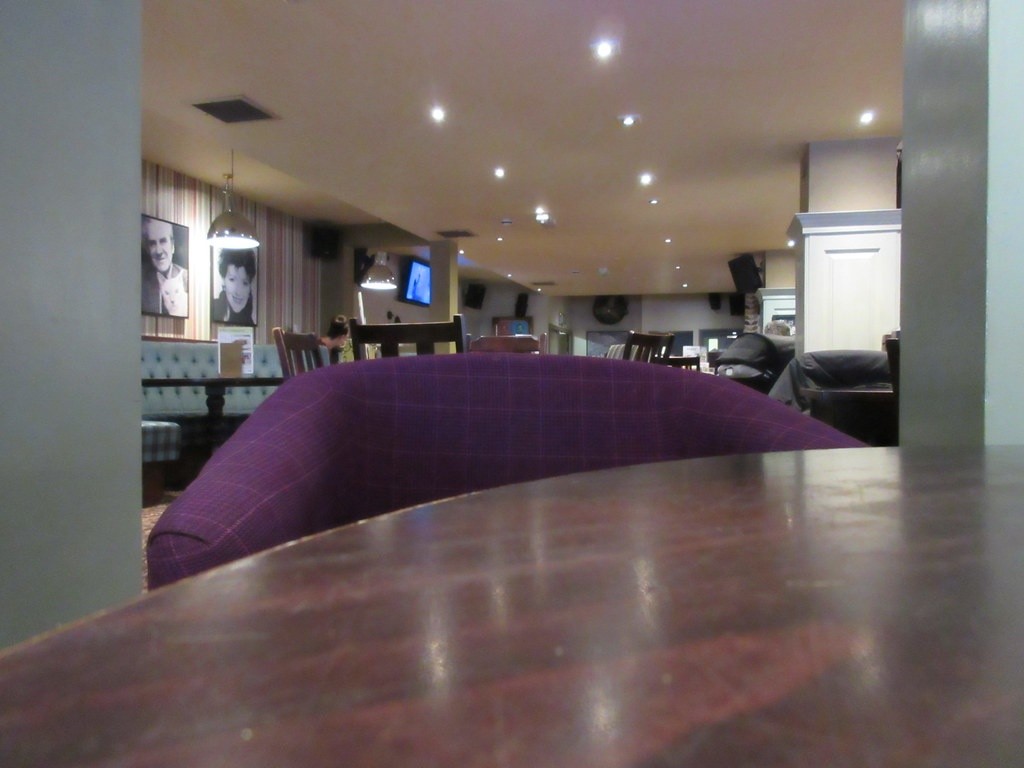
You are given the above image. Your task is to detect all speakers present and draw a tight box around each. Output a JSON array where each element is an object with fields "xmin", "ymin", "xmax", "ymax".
[
  {"xmin": 728, "ymin": 254, "xmax": 763, "ymax": 293},
  {"xmin": 312, "ymin": 229, "xmax": 339, "ymax": 259},
  {"xmin": 464, "ymin": 285, "xmax": 485, "ymax": 311}
]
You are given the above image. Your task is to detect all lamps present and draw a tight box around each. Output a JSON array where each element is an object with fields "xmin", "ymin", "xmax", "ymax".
[
  {"xmin": 360, "ymin": 251, "xmax": 398, "ymax": 291},
  {"xmin": 206, "ymin": 150, "xmax": 260, "ymax": 250}
]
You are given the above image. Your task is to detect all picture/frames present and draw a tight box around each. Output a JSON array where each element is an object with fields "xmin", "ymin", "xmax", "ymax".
[{"xmin": 491, "ymin": 317, "xmax": 533, "ymax": 338}]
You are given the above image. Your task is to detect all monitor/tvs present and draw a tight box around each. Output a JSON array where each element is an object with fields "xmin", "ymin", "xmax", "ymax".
[{"xmin": 401, "ymin": 259, "xmax": 431, "ymax": 306}]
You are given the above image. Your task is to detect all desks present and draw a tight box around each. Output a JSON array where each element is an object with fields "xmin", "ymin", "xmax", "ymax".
[{"xmin": 1, "ymin": 446, "xmax": 1024, "ymax": 768}]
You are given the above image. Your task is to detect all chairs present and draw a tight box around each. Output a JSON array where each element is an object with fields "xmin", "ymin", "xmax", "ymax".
[
  {"xmin": 146, "ymin": 312, "xmax": 901, "ymax": 589},
  {"xmin": 142, "ymin": 419, "xmax": 181, "ymax": 500}
]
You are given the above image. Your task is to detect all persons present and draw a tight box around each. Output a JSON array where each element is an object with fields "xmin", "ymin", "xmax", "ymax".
[
  {"xmin": 142, "ymin": 218, "xmax": 188, "ymax": 317},
  {"xmin": 212, "ymin": 248, "xmax": 256, "ymax": 327},
  {"xmin": 412, "ymin": 274, "xmax": 422, "ymax": 301},
  {"xmin": 312, "ymin": 315, "xmax": 349, "ymax": 367},
  {"xmin": 764, "ymin": 320, "xmax": 790, "ymax": 336}
]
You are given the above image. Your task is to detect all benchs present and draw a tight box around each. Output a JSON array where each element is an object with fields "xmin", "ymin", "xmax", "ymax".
[{"xmin": 138, "ymin": 332, "xmax": 330, "ymax": 420}]
